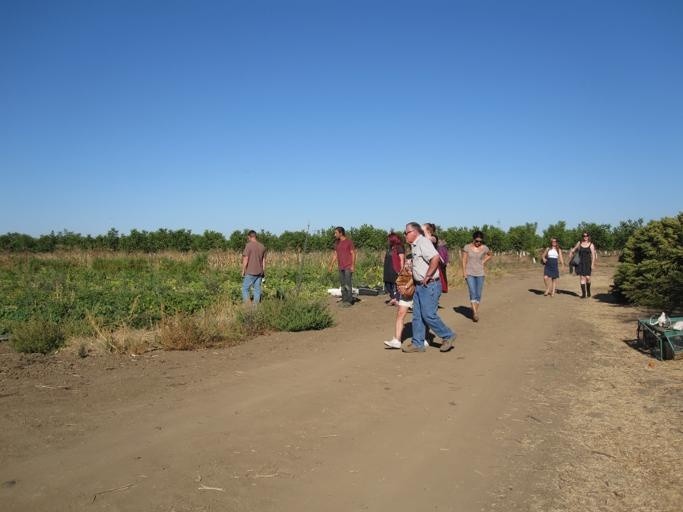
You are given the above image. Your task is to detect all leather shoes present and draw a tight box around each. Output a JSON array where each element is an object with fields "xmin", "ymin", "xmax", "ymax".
[{"xmin": 544, "ymin": 288, "xmax": 555, "ymax": 297}]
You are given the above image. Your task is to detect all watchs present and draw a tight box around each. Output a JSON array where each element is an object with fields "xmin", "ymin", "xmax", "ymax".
[{"xmin": 568, "ymin": 231, "xmax": 596, "ymax": 298}]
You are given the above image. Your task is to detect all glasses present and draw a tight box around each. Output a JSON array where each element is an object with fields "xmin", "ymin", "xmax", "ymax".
[
  {"xmin": 476, "ymin": 240, "xmax": 482, "ymax": 243},
  {"xmin": 582, "ymin": 235, "xmax": 589, "ymax": 237}
]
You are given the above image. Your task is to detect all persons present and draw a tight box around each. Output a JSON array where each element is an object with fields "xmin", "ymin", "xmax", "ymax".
[
  {"xmin": 541, "ymin": 237, "xmax": 565, "ymax": 297},
  {"xmin": 384, "ymin": 242, "xmax": 430, "ymax": 348},
  {"xmin": 240, "ymin": 230, "xmax": 268, "ymax": 305},
  {"xmin": 400, "ymin": 222, "xmax": 457, "ymax": 353},
  {"xmin": 382, "ymin": 233, "xmax": 405, "ymax": 305},
  {"xmin": 461, "ymin": 231, "xmax": 494, "ymax": 323},
  {"xmin": 408, "ymin": 223, "xmax": 439, "ymax": 310},
  {"xmin": 435, "ymin": 238, "xmax": 448, "ymax": 293},
  {"xmin": 327, "ymin": 226, "xmax": 356, "ymax": 308}
]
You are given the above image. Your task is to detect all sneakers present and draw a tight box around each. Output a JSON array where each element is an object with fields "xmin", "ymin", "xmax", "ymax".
[
  {"xmin": 472, "ymin": 313, "xmax": 479, "ymax": 322},
  {"xmin": 440, "ymin": 336, "xmax": 456, "ymax": 352},
  {"xmin": 403, "ymin": 334, "xmax": 433, "ymax": 352},
  {"xmin": 384, "ymin": 339, "xmax": 401, "ymax": 348}
]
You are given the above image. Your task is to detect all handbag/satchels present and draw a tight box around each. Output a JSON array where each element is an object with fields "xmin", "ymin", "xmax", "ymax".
[
  {"xmin": 396, "ymin": 265, "xmax": 415, "ymax": 297},
  {"xmin": 570, "ymin": 252, "xmax": 580, "ymax": 265},
  {"xmin": 541, "ymin": 255, "xmax": 547, "ymax": 265}
]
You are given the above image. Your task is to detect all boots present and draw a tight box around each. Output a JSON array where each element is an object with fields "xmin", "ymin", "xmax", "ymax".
[{"xmin": 581, "ymin": 283, "xmax": 590, "ymax": 298}]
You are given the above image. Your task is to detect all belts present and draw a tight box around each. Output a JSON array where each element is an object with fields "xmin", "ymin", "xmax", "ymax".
[{"xmin": 414, "ymin": 277, "xmax": 439, "ymax": 285}]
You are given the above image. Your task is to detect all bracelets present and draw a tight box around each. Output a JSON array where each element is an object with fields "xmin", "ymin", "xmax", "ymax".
[{"xmin": 426, "ymin": 275, "xmax": 431, "ymax": 277}]
